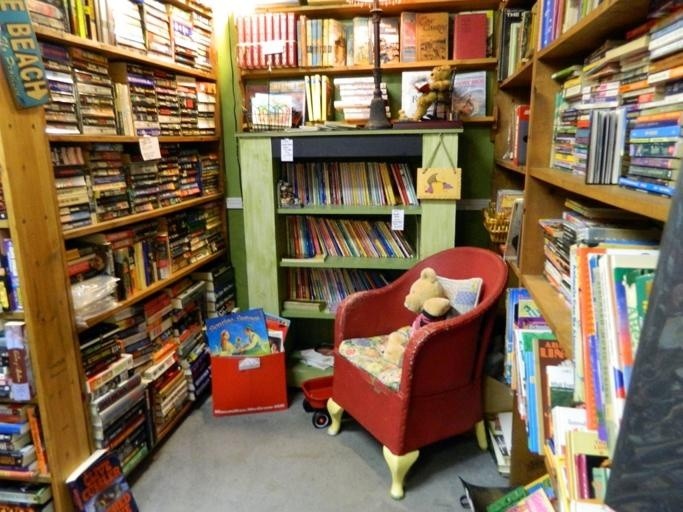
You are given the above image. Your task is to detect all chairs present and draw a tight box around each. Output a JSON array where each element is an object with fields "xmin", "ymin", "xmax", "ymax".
[{"xmin": 321, "ymin": 247, "xmax": 505, "ymax": 501}]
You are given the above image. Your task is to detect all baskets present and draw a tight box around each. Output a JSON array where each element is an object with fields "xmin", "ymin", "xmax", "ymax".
[{"xmin": 246, "ymin": 112, "xmax": 301, "ymax": 132}]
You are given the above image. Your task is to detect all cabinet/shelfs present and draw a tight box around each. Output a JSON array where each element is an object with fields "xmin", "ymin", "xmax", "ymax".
[
  {"xmin": 483, "ymin": 0, "xmax": 681, "ymax": 510},
  {"xmin": 232, "ymin": 0, "xmax": 498, "ymax": 319},
  {"xmin": 0, "ymin": 0, "xmax": 224, "ymax": 510}
]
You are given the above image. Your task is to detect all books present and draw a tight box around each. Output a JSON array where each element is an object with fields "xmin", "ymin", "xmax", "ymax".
[
  {"xmin": 0, "ymin": 233, "xmax": 55, "ymax": 512},
  {"xmin": 282, "ymin": 163, "xmax": 420, "ymax": 311},
  {"xmin": 236, "ymin": 10, "xmax": 494, "ymax": 128},
  {"xmin": 26, "ymin": 0, "xmax": 238, "ymax": 477},
  {"xmin": 489, "ymin": 0, "xmax": 683, "ymax": 512},
  {"xmin": 65, "ymin": 447, "xmax": 140, "ymax": 512}
]
